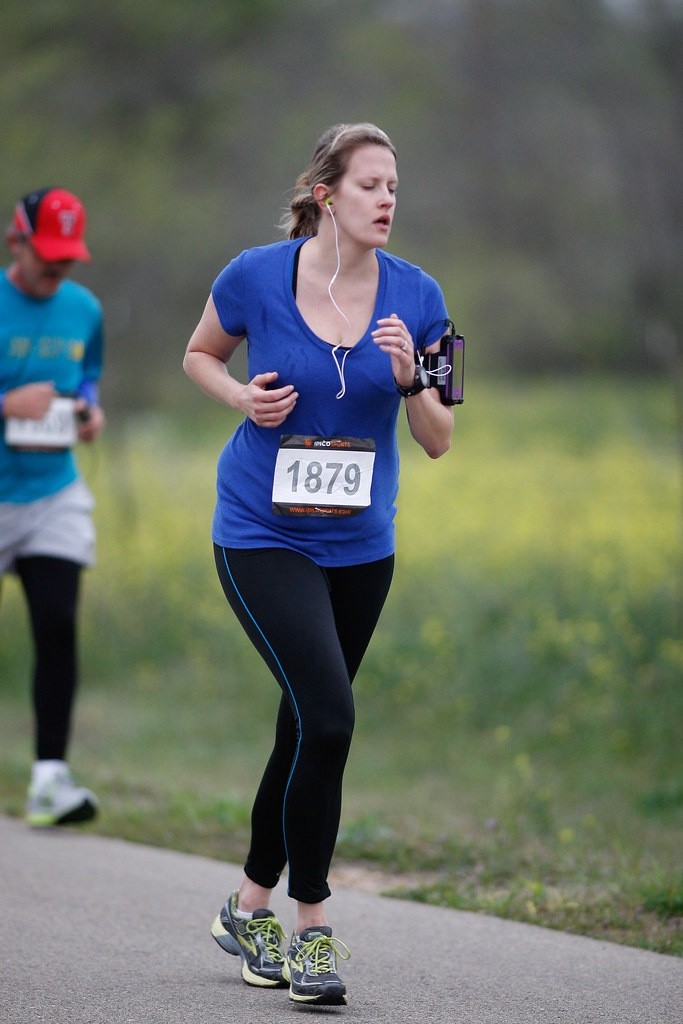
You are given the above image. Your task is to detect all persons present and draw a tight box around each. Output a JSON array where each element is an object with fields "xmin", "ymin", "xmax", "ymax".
[
  {"xmin": 0, "ymin": 189, "xmax": 109, "ymax": 828},
  {"xmin": 176, "ymin": 116, "xmax": 459, "ymax": 1004}
]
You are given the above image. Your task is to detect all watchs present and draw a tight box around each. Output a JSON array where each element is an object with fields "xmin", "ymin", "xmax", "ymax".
[{"xmin": 391, "ymin": 365, "xmax": 431, "ymax": 398}]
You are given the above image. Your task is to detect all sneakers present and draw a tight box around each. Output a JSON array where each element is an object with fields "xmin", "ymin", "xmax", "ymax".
[
  {"xmin": 209, "ymin": 893, "xmax": 289, "ymax": 990},
  {"xmin": 281, "ymin": 926, "xmax": 350, "ymax": 1005},
  {"xmin": 24, "ymin": 760, "xmax": 100, "ymax": 832}
]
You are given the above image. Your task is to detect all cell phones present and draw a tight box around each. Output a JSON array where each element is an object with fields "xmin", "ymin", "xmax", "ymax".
[{"xmin": 444, "ymin": 335, "xmax": 465, "ymax": 400}]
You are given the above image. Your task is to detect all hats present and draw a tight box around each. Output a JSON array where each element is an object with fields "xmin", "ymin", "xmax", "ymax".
[{"xmin": 13, "ymin": 188, "xmax": 92, "ymax": 267}]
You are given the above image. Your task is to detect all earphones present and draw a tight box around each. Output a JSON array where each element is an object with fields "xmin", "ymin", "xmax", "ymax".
[{"xmin": 326, "ymin": 198, "xmax": 332, "ymax": 210}]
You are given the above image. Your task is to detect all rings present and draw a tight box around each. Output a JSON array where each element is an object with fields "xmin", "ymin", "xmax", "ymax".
[{"xmin": 399, "ymin": 341, "xmax": 408, "ymax": 351}]
